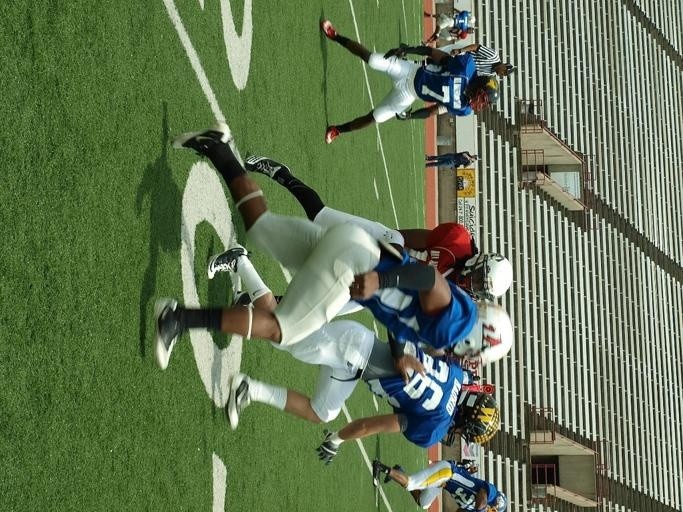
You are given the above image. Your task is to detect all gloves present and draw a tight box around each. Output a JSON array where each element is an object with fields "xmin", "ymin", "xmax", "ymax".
[
  {"xmin": 383, "ymin": 43, "xmax": 409, "ymax": 61},
  {"xmin": 396, "ymin": 108, "xmax": 412, "ymax": 121}
]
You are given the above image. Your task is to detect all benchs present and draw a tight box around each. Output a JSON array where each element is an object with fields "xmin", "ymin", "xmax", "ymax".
[{"xmin": 455, "ymin": 1, "xmax": 683, "ymax": 512}]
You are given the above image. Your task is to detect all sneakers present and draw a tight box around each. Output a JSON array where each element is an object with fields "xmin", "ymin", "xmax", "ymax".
[
  {"xmin": 207, "ymin": 243, "xmax": 248, "ymax": 280},
  {"xmin": 384, "ymin": 464, "xmax": 406, "ymax": 484},
  {"xmin": 225, "ymin": 372, "xmax": 252, "ymax": 430},
  {"xmin": 244, "ymin": 155, "xmax": 292, "ymax": 181},
  {"xmin": 324, "ymin": 125, "xmax": 341, "ymax": 146},
  {"xmin": 318, "ymin": 20, "xmax": 338, "ymax": 42},
  {"xmin": 315, "ymin": 426, "xmax": 338, "ymax": 467},
  {"xmin": 168, "ymin": 122, "xmax": 232, "ymax": 154},
  {"xmin": 152, "ymin": 295, "xmax": 182, "ymax": 372},
  {"xmin": 372, "ymin": 459, "xmax": 381, "ymax": 487}
]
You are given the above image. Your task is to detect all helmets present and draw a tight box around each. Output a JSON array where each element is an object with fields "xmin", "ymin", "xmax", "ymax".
[
  {"xmin": 467, "ymin": 75, "xmax": 500, "ymax": 107},
  {"xmin": 452, "ymin": 298, "xmax": 515, "ymax": 367},
  {"xmin": 451, "ymin": 387, "xmax": 501, "ymax": 446},
  {"xmin": 460, "ymin": 251, "xmax": 514, "ymax": 300}
]
